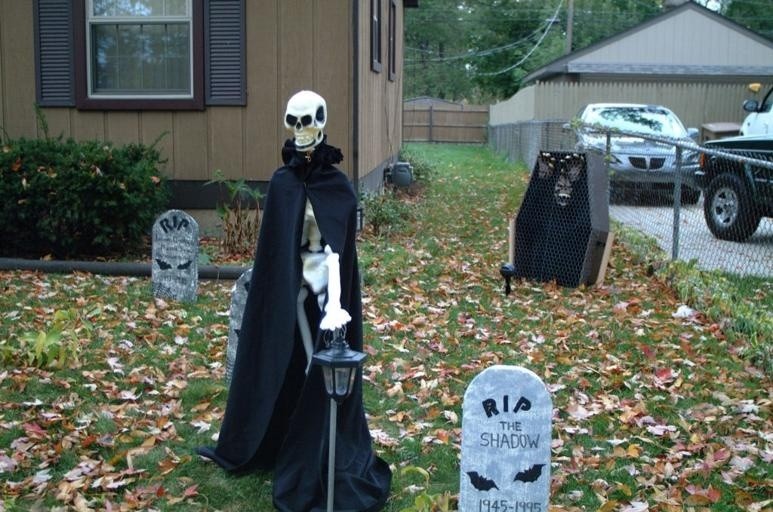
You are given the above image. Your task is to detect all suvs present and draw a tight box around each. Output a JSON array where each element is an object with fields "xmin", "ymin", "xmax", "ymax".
[{"xmin": 695, "ymin": 134, "xmax": 772, "ymax": 241}]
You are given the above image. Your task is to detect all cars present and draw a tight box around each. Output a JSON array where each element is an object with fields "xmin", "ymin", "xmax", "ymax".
[
  {"xmin": 737, "ymin": 84, "xmax": 773, "ymax": 145},
  {"xmin": 561, "ymin": 102, "xmax": 704, "ymax": 205}
]
